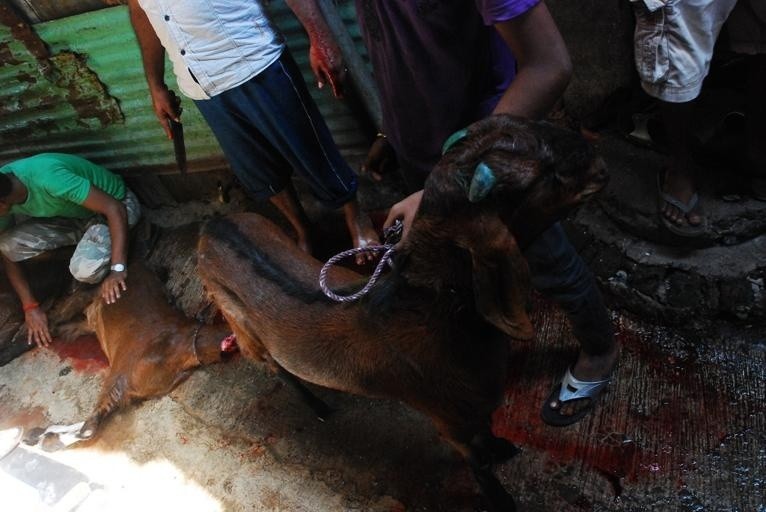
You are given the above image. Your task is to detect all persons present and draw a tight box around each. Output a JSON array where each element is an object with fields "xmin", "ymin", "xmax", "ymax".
[
  {"xmin": 0, "ymin": 151, "xmax": 143, "ymax": 352},
  {"xmin": 346, "ymin": 1, "xmax": 629, "ymax": 428},
  {"xmin": 123, "ymin": 0, "xmax": 385, "ymax": 274},
  {"xmin": 633, "ymin": 2, "xmax": 765, "ymax": 239}
]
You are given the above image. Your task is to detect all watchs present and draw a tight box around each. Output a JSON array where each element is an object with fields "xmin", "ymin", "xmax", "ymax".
[{"xmin": 109, "ymin": 263, "xmax": 128, "ymax": 272}]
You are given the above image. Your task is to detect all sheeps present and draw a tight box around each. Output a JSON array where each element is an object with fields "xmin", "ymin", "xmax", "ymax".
[
  {"xmin": 0, "ymin": 259, "xmax": 241, "ymax": 440},
  {"xmin": 196, "ymin": 113, "xmax": 612, "ymax": 512}
]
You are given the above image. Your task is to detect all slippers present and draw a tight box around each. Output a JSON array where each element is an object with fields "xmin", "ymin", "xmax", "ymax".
[
  {"xmin": 656, "ymin": 166, "xmax": 708, "ymax": 237},
  {"xmin": 540, "ymin": 358, "xmax": 610, "ymax": 426}
]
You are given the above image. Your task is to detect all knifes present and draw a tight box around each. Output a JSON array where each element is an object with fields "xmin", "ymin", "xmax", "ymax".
[{"xmin": 166, "ymin": 89, "xmax": 189, "ymax": 177}]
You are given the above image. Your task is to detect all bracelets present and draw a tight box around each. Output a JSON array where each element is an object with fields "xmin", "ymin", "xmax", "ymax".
[{"xmin": 22, "ymin": 302, "xmax": 40, "ymax": 311}]
